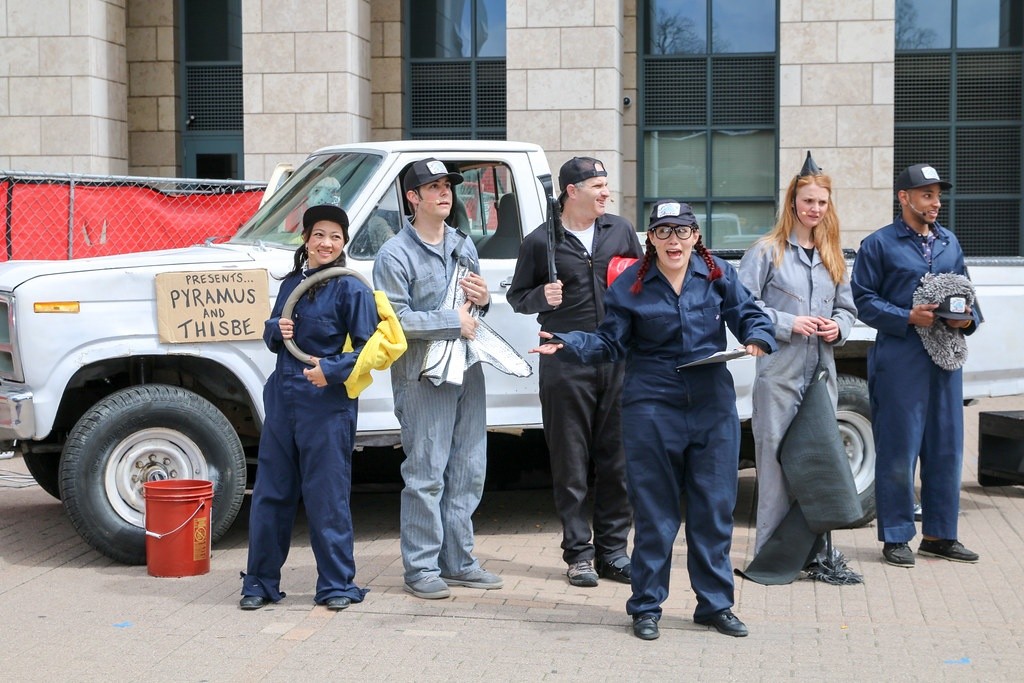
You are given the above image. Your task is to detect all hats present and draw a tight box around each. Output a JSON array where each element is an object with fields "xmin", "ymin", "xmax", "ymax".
[
  {"xmin": 896, "ymin": 164, "xmax": 953, "ymax": 190},
  {"xmin": 557, "ymin": 157, "xmax": 607, "ymax": 208},
  {"xmin": 649, "ymin": 199, "xmax": 696, "ymax": 230},
  {"xmin": 912, "ymin": 271, "xmax": 976, "ymax": 372},
  {"xmin": 303, "ymin": 205, "xmax": 349, "ymax": 228},
  {"xmin": 403, "ymin": 158, "xmax": 464, "ymax": 194}
]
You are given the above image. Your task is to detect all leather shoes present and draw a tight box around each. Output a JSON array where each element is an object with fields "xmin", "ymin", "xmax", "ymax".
[
  {"xmin": 240, "ymin": 596, "xmax": 264, "ymax": 610},
  {"xmin": 633, "ymin": 616, "xmax": 659, "ymax": 640},
  {"xmin": 327, "ymin": 597, "xmax": 351, "ymax": 610},
  {"xmin": 711, "ymin": 607, "xmax": 749, "ymax": 637}
]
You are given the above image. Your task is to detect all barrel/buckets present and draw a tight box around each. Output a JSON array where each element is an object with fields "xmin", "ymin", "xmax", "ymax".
[
  {"xmin": 144, "ymin": 479, "xmax": 214, "ymax": 577},
  {"xmin": 607, "ymin": 256, "xmax": 639, "ymax": 287}
]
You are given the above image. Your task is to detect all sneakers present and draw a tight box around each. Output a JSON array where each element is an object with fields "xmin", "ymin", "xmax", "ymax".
[
  {"xmin": 403, "ymin": 576, "xmax": 450, "ymax": 599},
  {"xmin": 567, "ymin": 560, "xmax": 599, "ymax": 587},
  {"xmin": 918, "ymin": 539, "xmax": 979, "ymax": 563},
  {"xmin": 594, "ymin": 556, "xmax": 631, "ymax": 584},
  {"xmin": 882, "ymin": 543, "xmax": 915, "ymax": 568},
  {"xmin": 440, "ymin": 567, "xmax": 504, "ymax": 589}
]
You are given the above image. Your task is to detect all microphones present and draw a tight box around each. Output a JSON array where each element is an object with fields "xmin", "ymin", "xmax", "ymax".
[
  {"xmin": 611, "ymin": 198, "xmax": 614, "ymax": 203},
  {"xmin": 306, "ymin": 243, "xmax": 315, "ymax": 255},
  {"xmin": 801, "ymin": 212, "xmax": 806, "ymax": 215},
  {"xmin": 909, "ymin": 201, "xmax": 927, "ymax": 216},
  {"xmin": 422, "ymin": 200, "xmax": 440, "ymax": 204}
]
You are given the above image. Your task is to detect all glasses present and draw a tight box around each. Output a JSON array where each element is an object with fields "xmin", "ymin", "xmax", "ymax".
[{"xmin": 651, "ymin": 225, "xmax": 699, "ymax": 240}]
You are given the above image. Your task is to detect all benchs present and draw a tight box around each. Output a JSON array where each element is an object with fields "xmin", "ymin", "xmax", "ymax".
[{"xmin": 453, "ymin": 193, "xmax": 523, "ymax": 259}]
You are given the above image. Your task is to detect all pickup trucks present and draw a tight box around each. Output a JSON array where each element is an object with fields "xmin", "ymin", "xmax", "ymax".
[{"xmin": 1, "ymin": 138, "xmax": 1024, "ymax": 562}]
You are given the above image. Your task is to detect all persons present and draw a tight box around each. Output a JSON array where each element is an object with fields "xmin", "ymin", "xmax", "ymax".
[
  {"xmin": 238, "ymin": 205, "xmax": 381, "ymax": 611},
  {"xmin": 506, "ymin": 156, "xmax": 642, "ymax": 582},
  {"xmin": 851, "ymin": 164, "xmax": 981, "ymax": 565},
  {"xmin": 528, "ymin": 202, "xmax": 778, "ymax": 640},
  {"xmin": 374, "ymin": 158, "xmax": 507, "ymax": 597},
  {"xmin": 740, "ymin": 151, "xmax": 864, "ymax": 582}
]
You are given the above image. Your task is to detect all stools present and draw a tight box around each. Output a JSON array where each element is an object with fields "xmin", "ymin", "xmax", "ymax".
[{"xmin": 977, "ymin": 410, "xmax": 1024, "ymax": 489}]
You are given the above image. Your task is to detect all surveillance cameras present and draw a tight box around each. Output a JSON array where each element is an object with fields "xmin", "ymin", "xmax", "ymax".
[{"xmin": 623, "ymin": 97, "xmax": 631, "ymax": 107}]
